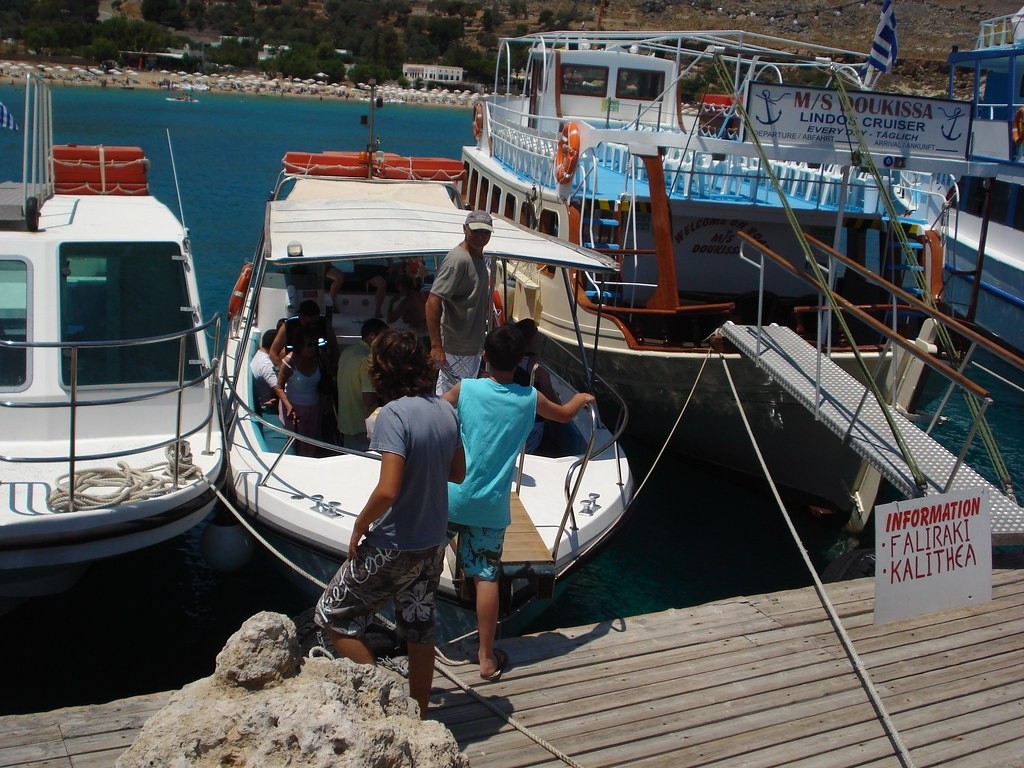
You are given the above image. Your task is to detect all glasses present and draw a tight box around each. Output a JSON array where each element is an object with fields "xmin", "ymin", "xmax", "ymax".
[{"xmin": 305, "ymin": 342, "xmax": 315, "ymax": 348}]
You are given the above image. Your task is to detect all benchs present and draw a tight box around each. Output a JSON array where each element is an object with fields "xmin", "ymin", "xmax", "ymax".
[{"xmin": 329, "ymin": 292, "xmax": 412, "ymax": 343}]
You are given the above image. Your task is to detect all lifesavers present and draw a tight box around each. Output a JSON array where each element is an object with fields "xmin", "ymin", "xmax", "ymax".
[
  {"xmin": 555, "ymin": 121, "xmax": 580, "ymax": 185},
  {"xmin": 473, "ymin": 105, "xmax": 482, "ymax": 137},
  {"xmin": 228, "ymin": 263, "xmax": 253, "ymax": 314},
  {"xmin": 1011, "ymin": 106, "xmax": 1024, "ymax": 144}
]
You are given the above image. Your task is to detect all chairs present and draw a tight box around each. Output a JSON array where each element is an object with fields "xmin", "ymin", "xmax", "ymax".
[{"xmin": 500, "ymin": 112, "xmax": 868, "ymax": 209}]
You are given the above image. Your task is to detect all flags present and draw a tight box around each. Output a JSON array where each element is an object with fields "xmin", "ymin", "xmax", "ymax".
[{"xmin": 861, "ymin": 0, "xmax": 898, "ymax": 77}]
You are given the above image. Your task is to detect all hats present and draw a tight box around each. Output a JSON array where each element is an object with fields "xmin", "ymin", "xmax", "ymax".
[
  {"xmin": 466, "ymin": 209, "xmax": 494, "ymax": 233},
  {"xmin": 295, "ymin": 300, "xmax": 320, "ymax": 317}
]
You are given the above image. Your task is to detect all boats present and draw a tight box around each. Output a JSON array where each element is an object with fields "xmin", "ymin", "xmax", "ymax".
[
  {"xmin": 919, "ymin": 8, "xmax": 1024, "ymax": 374},
  {"xmin": 472, "ymin": 37, "xmax": 1002, "ymax": 536},
  {"xmin": 218, "ymin": 78, "xmax": 635, "ymax": 607},
  {"xmin": 1, "ymin": 70, "xmax": 228, "ymax": 587}
]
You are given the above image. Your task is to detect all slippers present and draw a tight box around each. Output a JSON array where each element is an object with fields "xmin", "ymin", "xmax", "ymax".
[{"xmin": 480, "ymin": 648, "xmax": 508, "ymax": 682}]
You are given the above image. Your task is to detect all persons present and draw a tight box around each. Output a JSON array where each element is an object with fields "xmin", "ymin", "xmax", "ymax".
[
  {"xmin": 438, "ymin": 324, "xmax": 596, "ymax": 681},
  {"xmin": 312, "ymin": 328, "xmax": 479, "ymax": 724},
  {"xmin": 246, "ymin": 256, "xmax": 595, "ymax": 455},
  {"xmin": 425, "ymin": 210, "xmax": 495, "ymax": 402}
]
